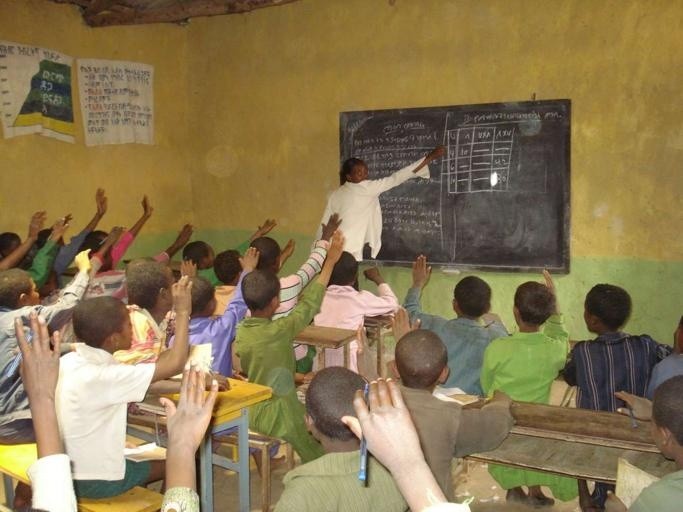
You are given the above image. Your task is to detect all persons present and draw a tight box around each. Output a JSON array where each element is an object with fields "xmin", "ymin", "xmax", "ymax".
[
  {"xmin": 0, "ymin": 187, "xmax": 347, "ymax": 511},
  {"xmin": 271, "ymin": 256, "xmax": 683, "ymax": 512},
  {"xmin": 311, "ymin": 145, "xmax": 447, "ymax": 292}
]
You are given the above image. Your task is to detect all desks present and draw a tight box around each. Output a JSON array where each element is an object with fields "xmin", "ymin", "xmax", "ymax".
[
  {"xmin": 126, "ymin": 317, "xmax": 397, "ymax": 512},
  {"xmin": 454, "ymin": 337, "xmax": 671, "ymax": 484}
]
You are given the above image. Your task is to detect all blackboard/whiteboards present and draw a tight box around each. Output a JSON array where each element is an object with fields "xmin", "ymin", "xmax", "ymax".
[{"xmin": 339, "ymin": 99, "xmax": 572, "ymax": 274}]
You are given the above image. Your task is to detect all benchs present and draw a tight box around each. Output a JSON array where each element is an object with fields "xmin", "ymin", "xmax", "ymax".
[{"xmin": 1, "ymin": 440, "xmax": 162, "ymax": 511}]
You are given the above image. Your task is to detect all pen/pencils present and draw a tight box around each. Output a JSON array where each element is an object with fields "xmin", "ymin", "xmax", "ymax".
[
  {"xmin": 626, "ymin": 401, "xmax": 637, "ymax": 428},
  {"xmin": 170, "ymin": 274, "xmax": 183, "ymax": 317},
  {"xmin": 7, "ymin": 330, "xmax": 34, "ymax": 377},
  {"xmin": 48, "ymin": 231, "xmax": 54, "ymax": 241},
  {"xmin": 359, "ymin": 383, "xmax": 369, "ymax": 481},
  {"xmin": 99, "ymin": 236, "xmax": 108, "ymax": 245}
]
